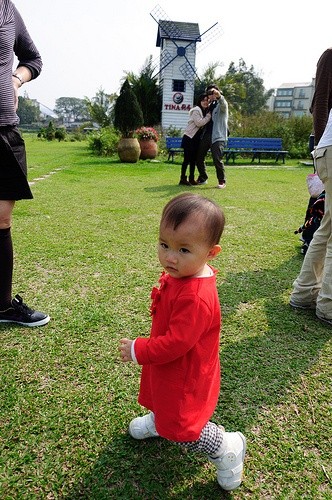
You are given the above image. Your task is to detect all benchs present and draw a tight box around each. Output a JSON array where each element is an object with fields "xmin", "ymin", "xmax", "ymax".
[
  {"xmin": 227, "ymin": 137, "xmax": 289, "ymax": 164},
  {"xmin": 165, "ymin": 136, "xmax": 232, "ymax": 165}
]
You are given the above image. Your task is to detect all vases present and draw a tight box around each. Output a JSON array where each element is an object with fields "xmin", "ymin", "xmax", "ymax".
[{"xmin": 140, "ymin": 139, "xmax": 158, "ymax": 159}]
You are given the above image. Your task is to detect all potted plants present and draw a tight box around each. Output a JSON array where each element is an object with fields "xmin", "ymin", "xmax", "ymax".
[
  {"xmin": 44, "ymin": 121, "xmax": 56, "ymax": 141},
  {"xmin": 113, "ymin": 77, "xmax": 144, "ymax": 163}
]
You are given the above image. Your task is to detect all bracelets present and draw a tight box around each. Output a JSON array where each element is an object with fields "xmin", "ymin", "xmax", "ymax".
[{"xmin": 12, "ymin": 73, "xmax": 24, "ymax": 84}]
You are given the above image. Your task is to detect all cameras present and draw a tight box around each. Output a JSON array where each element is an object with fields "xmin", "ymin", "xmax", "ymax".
[{"xmin": 208, "ymin": 91, "xmax": 213, "ymax": 95}]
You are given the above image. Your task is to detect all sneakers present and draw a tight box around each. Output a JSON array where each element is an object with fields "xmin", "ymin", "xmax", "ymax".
[{"xmin": 0, "ymin": 294, "xmax": 50, "ymax": 327}]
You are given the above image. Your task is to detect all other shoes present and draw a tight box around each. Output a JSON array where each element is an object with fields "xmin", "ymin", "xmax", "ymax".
[
  {"xmin": 129, "ymin": 413, "xmax": 160, "ymax": 438},
  {"xmin": 198, "ymin": 175, "xmax": 207, "ymax": 184},
  {"xmin": 218, "ymin": 182, "xmax": 226, "ymax": 188},
  {"xmin": 210, "ymin": 432, "xmax": 246, "ymax": 490},
  {"xmin": 289, "ymin": 300, "xmax": 315, "ymax": 309},
  {"xmin": 301, "ymin": 247, "xmax": 307, "ymax": 253},
  {"xmin": 317, "ymin": 314, "xmax": 332, "ymax": 324}
]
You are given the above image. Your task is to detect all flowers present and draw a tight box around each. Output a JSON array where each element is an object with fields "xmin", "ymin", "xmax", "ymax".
[{"xmin": 136, "ymin": 126, "xmax": 159, "ymax": 142}]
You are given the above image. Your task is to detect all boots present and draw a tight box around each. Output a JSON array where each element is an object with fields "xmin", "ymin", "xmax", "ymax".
[
  {"xmin": 179, "ymin": 175, "xmax": 190, "ymax": 185},
  {"xmin": 188, "ymin": 174, "xmax": 198, "ymax": 184}
]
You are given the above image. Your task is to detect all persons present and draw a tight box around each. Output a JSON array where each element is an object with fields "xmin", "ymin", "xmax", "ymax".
[
  {"xmin": 0, "ymin": 0, "xmax": 50, "ymax": 327},
  {"xmin": 179, "ymin": 95, "xmax": 211, "ymax": 186},
  {"xmin": 196, "ymin": 85, "xmax": 228, "ymax": 188},
  {"xmin": 120, "ymin": 193, "xmax": 247, "ymax": 490},
  {"xmin": 290, "ymin": 49, "xmax": 332, "ymax": 323}
]
